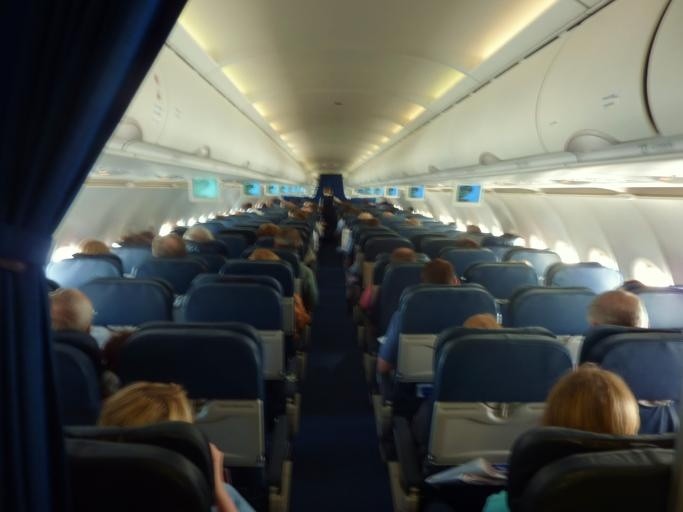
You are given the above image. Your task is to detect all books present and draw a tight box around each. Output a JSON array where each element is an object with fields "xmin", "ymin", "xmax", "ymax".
[{"xmin": 423, "ymin": 457, "xmax": 508, "ymax": 488}]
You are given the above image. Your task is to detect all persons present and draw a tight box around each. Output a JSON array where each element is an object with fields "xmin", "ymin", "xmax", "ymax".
[
  {"xmin": 319, "ymin": 187, "xmax": 341, "ymax": 231},
  {"xmin": 93, "ymin": 380, "xmax": 256, "ymax": 511},
  {"xmin": 480, "ymin": 362, "xmax": 640, "ymax": 512},
  {"xmin": 80, "ymin": 197, "xmax": 324, "ymax": 347},
  {"xmin": 621, "ymin": 277, "xmax": 653, "ymax": 292},
  {"xmin": 338, "ymin": 201, "xmax": 512, "ymax": 372},
  {"xmin": 394, "ymin": 311, "xmax": 506, "ymax": 443},
  {"xmin": 48, "ymin": 284, "xmax": 94, "ymax": 333},
  {"xmin": 560, "ymin": 289, "xmax": 648, "ymax": 370}
]
